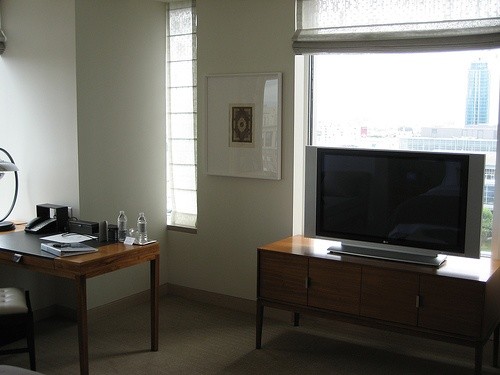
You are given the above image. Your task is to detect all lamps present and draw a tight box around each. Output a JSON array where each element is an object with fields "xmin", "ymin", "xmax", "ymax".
[{"xmin": 0, "ymin": 148, "xmax": 19, "ymax": 232}]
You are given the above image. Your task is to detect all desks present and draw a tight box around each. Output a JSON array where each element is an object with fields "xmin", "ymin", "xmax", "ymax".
[{"xmin": 0, "ymin": 222, "xmax": 160, "ymax": 375}]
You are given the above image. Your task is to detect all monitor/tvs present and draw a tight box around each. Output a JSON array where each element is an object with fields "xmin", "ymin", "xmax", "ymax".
[{"xmin": 305, "ymin": 144, "xmax": 485, "ymax": 267}]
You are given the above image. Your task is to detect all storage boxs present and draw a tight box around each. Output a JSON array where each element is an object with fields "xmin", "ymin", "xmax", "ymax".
[{"xmin": 67, "ymin": 219, "xmax": 99, "ymax": 235}]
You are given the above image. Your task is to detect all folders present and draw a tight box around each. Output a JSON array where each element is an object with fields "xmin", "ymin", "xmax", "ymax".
[{"xmin": 40, "ymin": 242, "xmax": 98, "ymax": 258}]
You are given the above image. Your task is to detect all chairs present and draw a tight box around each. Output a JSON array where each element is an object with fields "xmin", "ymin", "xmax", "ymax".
[{"xmin": 0, "ymin": 287, "xmax": 36, "ymax": 371}]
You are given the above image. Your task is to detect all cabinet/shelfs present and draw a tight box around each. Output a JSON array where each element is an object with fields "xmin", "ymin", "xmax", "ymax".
[{"xmin": 255, "ymin": 235, "xmax": 500, "ymax": 375}]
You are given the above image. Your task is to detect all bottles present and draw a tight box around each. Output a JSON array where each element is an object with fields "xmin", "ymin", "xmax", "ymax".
[
  {"xmin": 118, "ymin": 211, "xmax": 128, "ymax": 242},
  {"xmin": 137, "ymin": 212, "xmax": 148, "ymax": 245}
]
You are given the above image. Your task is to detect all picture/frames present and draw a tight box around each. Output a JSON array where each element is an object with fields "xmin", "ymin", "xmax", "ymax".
[{"xmin": 204, "ymin": 71, "xmax": 283, "ymax": 180}]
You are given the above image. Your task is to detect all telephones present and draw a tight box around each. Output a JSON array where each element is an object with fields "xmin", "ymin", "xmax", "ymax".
[{"xmin": 24, "ymin": 214, "xmax": 56, "ymax": 235}]
